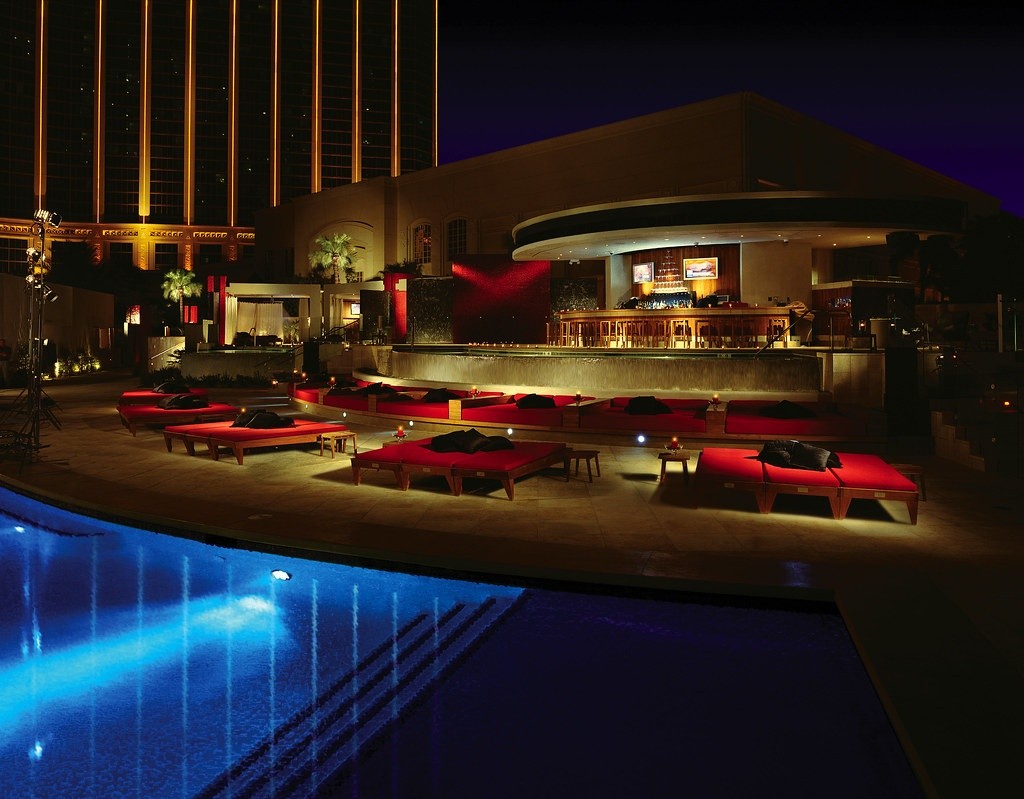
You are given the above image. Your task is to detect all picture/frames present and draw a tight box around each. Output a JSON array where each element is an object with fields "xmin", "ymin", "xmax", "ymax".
[{"xmin": 683, "ymin": 257, "xmax": 718, "ymax": 280}]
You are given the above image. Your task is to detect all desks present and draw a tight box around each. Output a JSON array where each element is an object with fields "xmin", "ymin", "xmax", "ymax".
[
  {"xmin": 196, "ymin": 411, "xmax": 226, "ymax": 425},
  {"xmin": 888, "ymin": 464, "xmax": 927, "ymax": 501},
  {"xmin": 560, "ymin": 451, "xmax": 601, "ymax": 483},
  {"xmin": 660, "ymin": 450, "xmax": 691, "ymax": 486},
  {"xmin": 320, "ymin": 429, "xmax": 357, "ymax": 458}
]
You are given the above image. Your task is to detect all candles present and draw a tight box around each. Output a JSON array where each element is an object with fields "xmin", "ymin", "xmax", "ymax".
[
  {"xmin": 299, "ymin": 372, "xmax": 309, "ymax": 381},
  {"xmin": 668, "ymin": 434, "xmax": 681, "ymax": 449},
  {"xmin": 239, "ymin": 401, "xmax": 246, "ymax": 414},
  {"xmin": 328, "ymin": 375, "xmax": 336, "ymax": 391},
  {"xmin": 394, "ymin": 423, "xmax": 406, "ymax": 440},
  {"xmin": 271, "ymin": 380, "xmax": 279, "ymax": 390}
]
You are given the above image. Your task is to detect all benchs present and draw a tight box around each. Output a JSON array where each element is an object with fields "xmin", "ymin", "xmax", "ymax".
[{"xmin": 113, "ymin": 376, "xmax": 922, "ymax": 526}]
